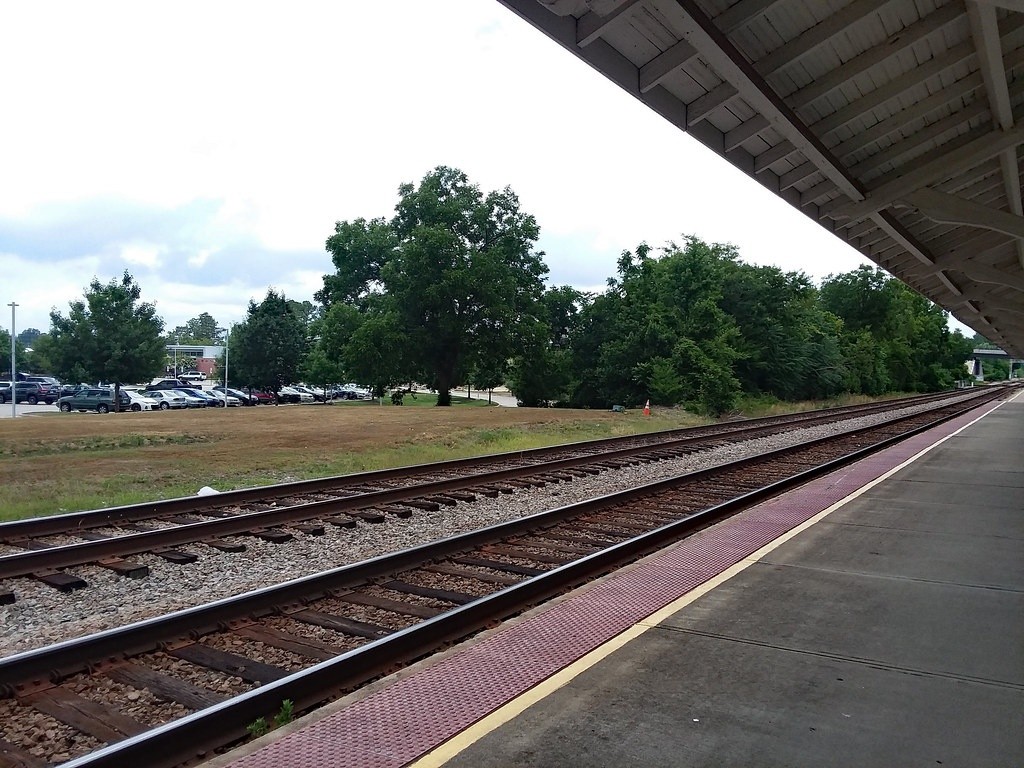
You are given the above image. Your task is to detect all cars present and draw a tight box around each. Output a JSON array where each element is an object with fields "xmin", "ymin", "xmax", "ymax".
[
  {"xmin": 217, "ymin": 388, "xmax": 258, "ymax": 406},
  {"xmin": 125, "ymin": 390, "xmax": 159, "ymax": 411},
  {"xmin": 0, "ymin": 376, "xmax": 92, "ymax": 405},
  {"xmin": 243, "ymin": 383, "xmax": 375, "ymax": 402},
  {"xmin": 141, "ymin": 391, "xmax": 188, "ymax": 410},
  {"xmin": 172, "ymin": 390, "xmax": 206, "ymax": 408},
  {"xmin": 204, "ymin": 390, "xmax": 240, "ymax": 407}
]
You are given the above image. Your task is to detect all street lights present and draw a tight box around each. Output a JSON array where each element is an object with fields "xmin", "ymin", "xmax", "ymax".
[
  {"xmin": 7, "ymin": 302, "xmax": 20, "ymax": 418},
  {"xmin": 221, "ymin": 328, "xmax": 229, "ymax": 408}
]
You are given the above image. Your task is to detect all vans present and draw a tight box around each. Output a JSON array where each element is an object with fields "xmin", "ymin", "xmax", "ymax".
[
  {"xmin": 177, "ymin": 371, "xmax": 207, "ymax": 381},
  {"xmin": 172, "ymin": 388, "xmax": 219, "ymax": 407}
]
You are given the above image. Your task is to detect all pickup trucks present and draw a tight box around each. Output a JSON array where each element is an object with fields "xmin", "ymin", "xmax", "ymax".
[{"xmin": 145, "ymin": 380, "xmax": 202, "ymax": 390}]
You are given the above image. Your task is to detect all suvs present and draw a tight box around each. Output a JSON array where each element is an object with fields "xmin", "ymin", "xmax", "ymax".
[{"xmin": 56, "ymin": 389, "xmax": 130, "ymax": 414}]
